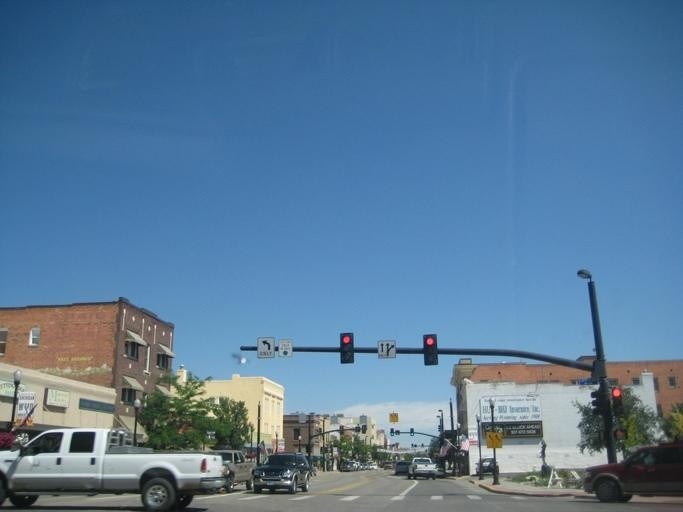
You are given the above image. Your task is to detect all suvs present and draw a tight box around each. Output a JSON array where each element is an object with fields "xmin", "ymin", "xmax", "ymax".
[
  {"xmin": 249, "ymin": 453, "xmax": 312, "ymax": 494},
  {"xmin": 578, "ymin": 441, "xmax": 683, "ymax": 505}
]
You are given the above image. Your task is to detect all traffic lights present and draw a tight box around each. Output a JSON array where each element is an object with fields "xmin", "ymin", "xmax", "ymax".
[
  {"xmin": 390, "ymin": 428, "xmax": 394, "ymax": 436},
  {"xmin": 614, "ymin": 429, "xmax": 628, "ymax": 441},
  {"xmin": 338, "ymin": 333, "xmax": 354, "ymax": 365},
  {"xmin": 409, "ymin": 428, "xmax": 414, "ymax": 436},
  {"xmin": 422, "ymin": 333, "xmax": 438, "ymax": 367},
  {"xmin": 611, "ymin": 384, "xmax": 623, "ymax": 417},
  {"xmin": 589, "ymin": 388, "xmax": 601, "ymax": 416}
]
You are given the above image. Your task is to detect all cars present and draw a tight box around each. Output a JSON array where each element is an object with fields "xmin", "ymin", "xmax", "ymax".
[
  {"xmin": 337, "ymin": 457, "xmax": 411, "ymax": 474},
  {"xmin": 474, "ymin": 457, "xmax": 499, "ymax": 475}
]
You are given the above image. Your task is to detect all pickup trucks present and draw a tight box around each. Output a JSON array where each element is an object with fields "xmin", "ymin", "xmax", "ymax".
[
  {"xmin": 206, "ymin": 447, "xmax": 257, "ymax": 493},
  {"xmin": 406, "ymin": 456, "xmax": 437, "ymax": 480},
  {"xmin": 0, "ymin": 425, "xmax": 232, "ymax": 512}
]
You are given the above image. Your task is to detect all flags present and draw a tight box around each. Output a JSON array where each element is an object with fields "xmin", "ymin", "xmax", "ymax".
[
  {"xmin": 440, "ymin": 439, "xmax": 451, "ymax": 458},
  {"xmin": 459, "ymin": 435, "xmax": 470, "ymax": 452}
]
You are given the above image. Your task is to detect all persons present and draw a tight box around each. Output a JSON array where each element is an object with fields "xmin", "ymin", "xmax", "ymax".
[{"xmin": 0, "ymin": 422, "xmax": 14, "ymax": 451}]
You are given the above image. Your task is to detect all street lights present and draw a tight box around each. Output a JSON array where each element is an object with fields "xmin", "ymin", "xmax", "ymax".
[
  {"xmin": 488, "ymin": 395, "xmax": 501, "ymax": 486},
  {"xmin": 321, "ymin": 416, "xmax": 328, "ymax": 472},
  {"xmin": 572, "ymin": 264, "xmax": 619, "ymax": 465},
  {"xmin": 364, "ymin": 434, "xmax": 368, "ymax": 464},
  {"xmin": 131, "ymin": 396, "xmax": 142, "ymax": 446},
  {"xmin": 298, "ymin": 434, "xmax": 302, "ymax": 453},
  {"xmin": 474, "ymin": 412, "xmax": 484, "ymax": 482},
  {"xmin": 7, "ymin": 367, "xmax": 22, "ymax": 432},
  {"xmin": 274, "ymin": 429, "xmax": 280, "ymax": 453},
  {"xmin": 435, "ymin": 408, "xmax": 446, "ymax": 467}
]
